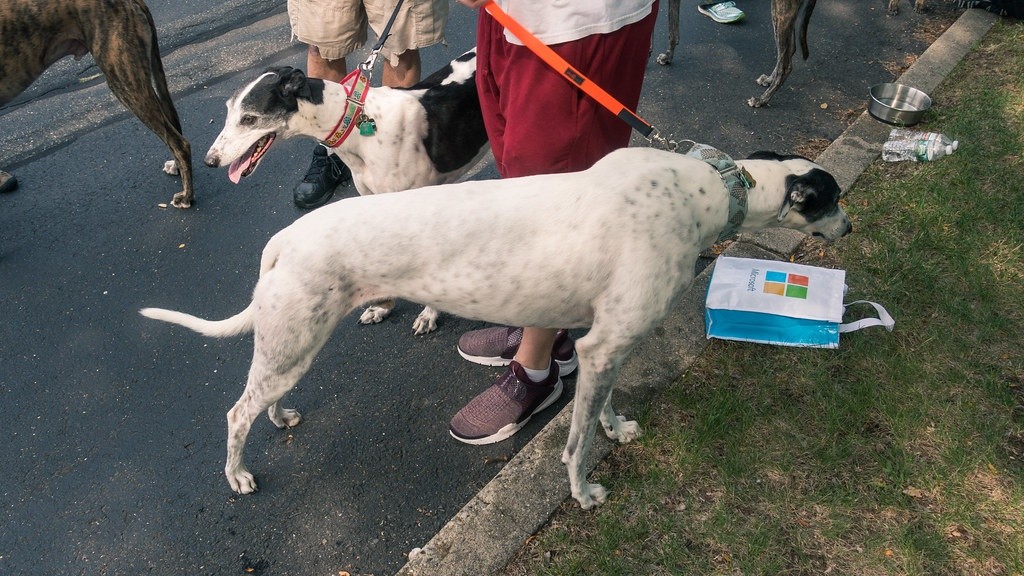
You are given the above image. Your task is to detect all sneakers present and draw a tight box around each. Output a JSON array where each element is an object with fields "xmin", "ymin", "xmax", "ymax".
[
  {"xmin": 698, "ymin": 0, "xmax": 744, "ymax": 23},
  {"xmin": 292, "ymin": 144, "xmax": 351, "ymax": 210},
  {"xmin": 457, "ymin": 324, "xmax": 579, "ymax": 377},
  {"xmin": 449, "ymin": 361, "xmax": 564, "ymax": 445}
]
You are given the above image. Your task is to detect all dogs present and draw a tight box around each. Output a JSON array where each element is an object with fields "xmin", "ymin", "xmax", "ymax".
[
  {"xmin": 202, "ymin": 41, "xmax": 490, "ymax": 337},
  {"xmin": 0, "ymin": 0, "xmax": 197, "ymax": 210},
  {"xmin": 135, "ymin": 145, "xmax": 853, "ymax": 511},
  {"xmin": 647, "ymin": 0, "xmax": 817, "ymax": 109},
  {"xmin": 886, "ymin": 0, "xmax": 926, "ymax": 17}
]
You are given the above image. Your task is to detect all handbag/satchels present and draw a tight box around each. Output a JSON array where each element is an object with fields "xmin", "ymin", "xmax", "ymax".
[{"xmin": 702, "ymin": 255, "xmax": 895, "ymax": 350}]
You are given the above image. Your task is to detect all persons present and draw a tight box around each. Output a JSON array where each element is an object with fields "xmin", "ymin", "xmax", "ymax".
[
  {"xmin": 287, "ymin": 0, "xmax": 453, "ymax": 209},
  {"xmin": 449, "ymin": 0, "xmax": 659, "ymax": 444}
]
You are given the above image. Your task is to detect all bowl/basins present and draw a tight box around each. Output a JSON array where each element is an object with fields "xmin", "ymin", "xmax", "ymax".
[{"xmin": 869, "ymin": 83, "xmax": 932, "ymax": 126}]
